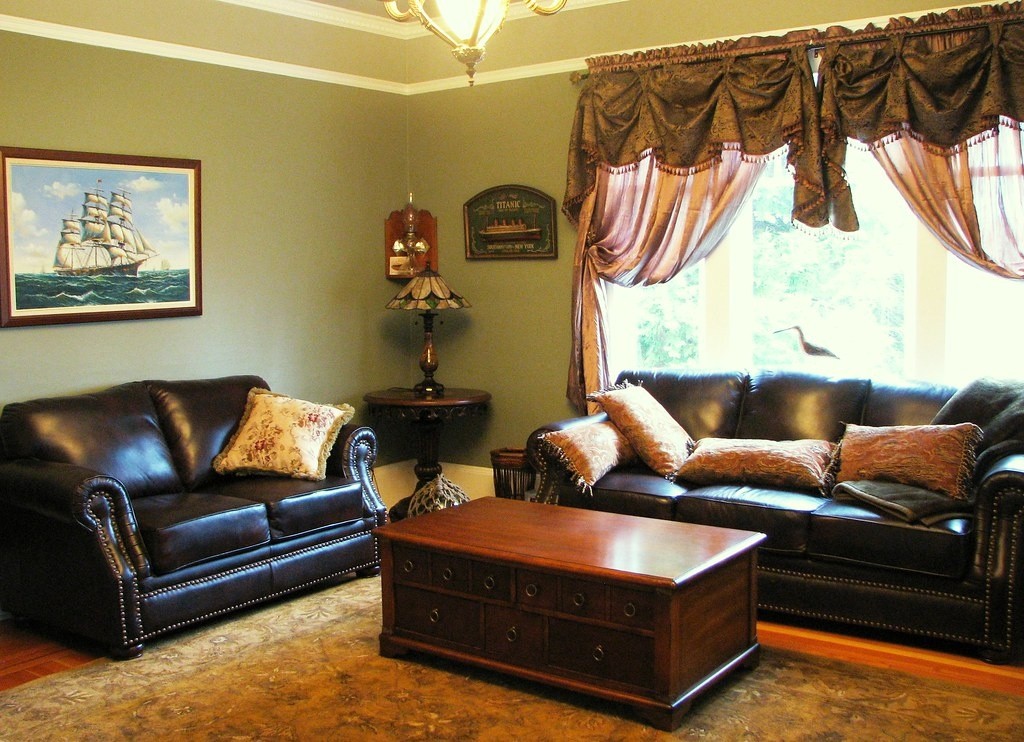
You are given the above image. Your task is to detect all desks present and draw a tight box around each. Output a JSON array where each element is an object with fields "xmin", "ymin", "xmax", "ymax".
[{"xmin": 362, "ymin": 388, "xmax": 493, "ymax": 523}]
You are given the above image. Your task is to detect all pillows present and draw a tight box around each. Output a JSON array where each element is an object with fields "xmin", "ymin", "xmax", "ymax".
[
  {"xmin": 213, "ymin": 386, "xmax": 356, "ymax": 483},
  {"xmin": 666, "ymin": 437, "xmax": 843, "ymax": 501},
  {"xmin": 834, "ymin": 422, "xmax": 985, "ymax": 501},
  {"xmin": 536, "ymin": 420, "xmax": 639, "ymax": 497},
  {"xmin": 584, "ymin": 380, "xmax": 696, "ymax": 482}
]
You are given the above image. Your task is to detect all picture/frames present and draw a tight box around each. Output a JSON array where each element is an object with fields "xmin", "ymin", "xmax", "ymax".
[
  {"xmin": 0, "ymin": 146, "xmax": 203, "ymax": 328},
  {"xmin": 462, "ymin": 182, "xmax": 556, "ymax": 260}
]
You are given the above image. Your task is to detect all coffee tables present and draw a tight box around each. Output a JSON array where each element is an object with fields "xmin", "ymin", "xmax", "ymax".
[{"xmin": 371, "ymin": 494, "xmax": 767, "ymax": 734}]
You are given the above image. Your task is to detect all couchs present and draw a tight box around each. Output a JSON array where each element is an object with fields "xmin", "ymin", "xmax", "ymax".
[
  {"xmin": 527, "ymin": 369, "xmax": 1024, "ymax": 665},
  {"xmin": 0, "ymin": 375, "xmax": 391, "ymax": 664}
]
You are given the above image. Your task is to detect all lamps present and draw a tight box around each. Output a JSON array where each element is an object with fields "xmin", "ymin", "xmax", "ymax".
[
  {"xmin": 376, "ymin": 0, "xmax": 568, "ymax": 88},
  {"xmin": 385, "ymin": 257, "xmax": 472, "ymax": 394}
]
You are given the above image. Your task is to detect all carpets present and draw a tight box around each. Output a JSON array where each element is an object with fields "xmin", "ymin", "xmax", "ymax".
[{"xmin": 0, "ymin": 570, "xmax": 1024, "ymax": 742}]
